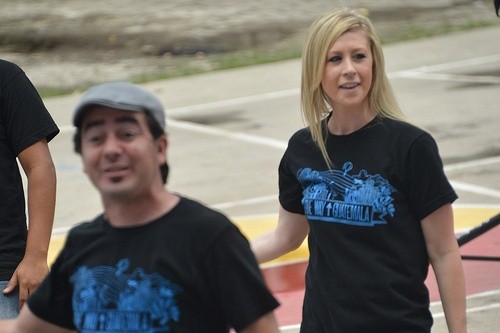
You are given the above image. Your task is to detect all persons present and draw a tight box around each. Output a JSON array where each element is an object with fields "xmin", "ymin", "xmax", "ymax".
[
  {"xmin": 246, "ymin": 5, "xmax": 469, "ymax": 333},
  {"xmin": 0, "ymin": 79, "xmax": 282, "ymax": 332},
  {"xmin": 0, "ymin": 59, "xmax": 59, "ymax": 322}
]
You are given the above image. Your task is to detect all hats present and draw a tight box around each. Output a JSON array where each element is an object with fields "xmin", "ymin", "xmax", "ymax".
[{"xmin": 73, "ymin": 83, "xmax": 164, "ymax": 133}]
